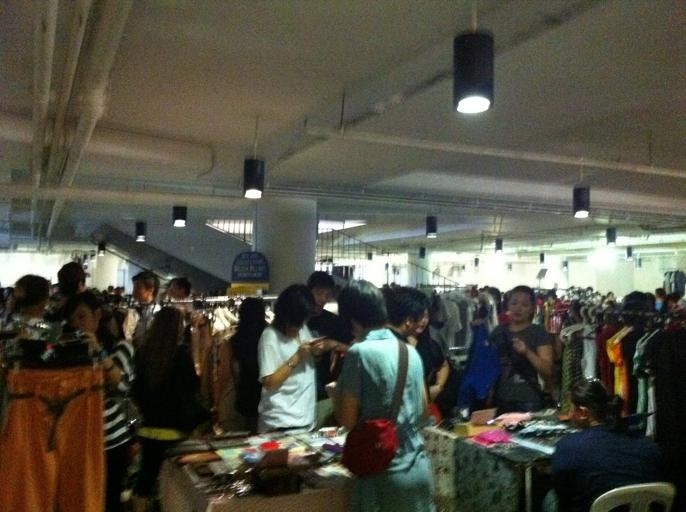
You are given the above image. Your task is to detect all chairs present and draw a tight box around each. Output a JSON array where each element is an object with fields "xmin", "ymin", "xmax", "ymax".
[{"xmin": 590, "ymin": 482, "xmax": 673, "ymax": 511}]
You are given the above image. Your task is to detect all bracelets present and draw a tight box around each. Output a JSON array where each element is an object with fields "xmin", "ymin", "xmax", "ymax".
[{"xmin": 104, "ymin": 363, "xmax": 114, "ymax": 372}]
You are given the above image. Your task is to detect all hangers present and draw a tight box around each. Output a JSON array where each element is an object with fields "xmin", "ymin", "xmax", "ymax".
[{"xmin": 561, "ymin": 304, "xmax": 686, "ymax": 348}]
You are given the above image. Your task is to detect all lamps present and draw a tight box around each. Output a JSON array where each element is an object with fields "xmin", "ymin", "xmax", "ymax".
[
  {"xmin": 83, "ymin": 254, "xmax": 88, "ymax": 267},
  {"xmin": 135, "ymin": 223, "xmax": 148, "ymax": 243},
  {"xmin": 424, "ymin": 215, "xmax": 437, "ymax": 239},
  {"xmin": 453, "ymin": 0, "xmax": 495, "ymax": 114},
  {"xmin": 99, "ymin": 242, "xmax": 105, "ymax": 256},
  {"xmin": 494, "ymin": 237, "xmax": 503, "ymax": 254},
  {"xmin": 242, "ymin": 116, "xmax": 265, "ymax": 200},
  {"xmin": 172, "ymin": 205, "xmax": 187, "ymax": 228},
  {"xmin": 572, "ymin": 153, "xmax": 589, "ymax": 220}
]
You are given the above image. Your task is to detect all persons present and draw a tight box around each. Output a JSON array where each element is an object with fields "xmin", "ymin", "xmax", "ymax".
[
  {"xmin": 475, "ymin": 288, "xmax": 502, "ymax": 318},
  {"xmin": 108, "ymin": 285, "xmax": 125, "ymax": 296},
  {"xmin": 671, "ymin": 293, "xmax": 680, "ymax": 304},
  {"xmin": 132, "ymin": 271, "xmax": 163, "ymax": 346},
  {"xmin": 654, "ymin": 288, "xmax": 666, "ymax": 311},
  {"xmin": 622, "ymin": 291, "xmax": 645, "ymax": 312},
  {"xmin": 470, "ymin": 285, "xmax": 554, "ymax": 407},
  {"xmin": 229, "ymin": 297, "xmax": 265, "ymax": 432},
  {"xmin": 121, "ymin": 287, "xmax": 125, "ymax": 297},
  {"xmin": 134, "ymin": 307, "xmax": 214, "ymax": 512},
  {"xmin": 307, "ymin": 270, "xmax": 353, "ymax": 402},
  {"xmin": 552, "ymin": 378, "xmax": 666, "ymax": 511},
  {"xmin": 326, "ymin": 279, "xmax": 434, "ymax": 512},
  {"xmin": 167, "ymin": 278, "xmax": 178, "ymax": 298},
  {"xmin": 176, "ymin": 278, "xmax": 191, "ymax": 299},
  {"xmin": 258, "ymin": 284, "xmax": 318, "ymax": 432},
  {"xmin": 0, "ymin": 274, "xmax": 61, "ymax": 367},
  {"xmin": 659, "ymin": 294, "xmax": 675, "ymax": 314},
  {"xmin": 58, "ymin": 263, "xmax": 87, "ymax": 301},
  {"xmin": 64, "ymin": 292, "xmax": 137, "ymax": 512},
  {"xmin": 408, "ymin": 299, "xmax": 451, "ymax": 404},
  {"xmin": 386, "ymin": 285, "xmax": 427, "ymax": 343},
  {"xmin": 645, "ymin": 291, "xmax": 656, "ymax": 312}
]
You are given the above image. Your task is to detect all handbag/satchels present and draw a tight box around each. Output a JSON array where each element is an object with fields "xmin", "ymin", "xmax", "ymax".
[{"xmin": 341, "ymin": 339, "xmax": 409, "ymax": 472}]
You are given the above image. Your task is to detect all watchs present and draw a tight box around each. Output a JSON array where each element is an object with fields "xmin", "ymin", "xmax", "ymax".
[
  {"xmin": 94, "ymin": 350, "xmax": 107, "ymax": 361},
  {"xmin": 434, "ymin": 384, "xmax": 445, "ymax": 391},
  {"xmin": 288, "ymin": 360, "xmax": 297, "ymax": 368}
]
